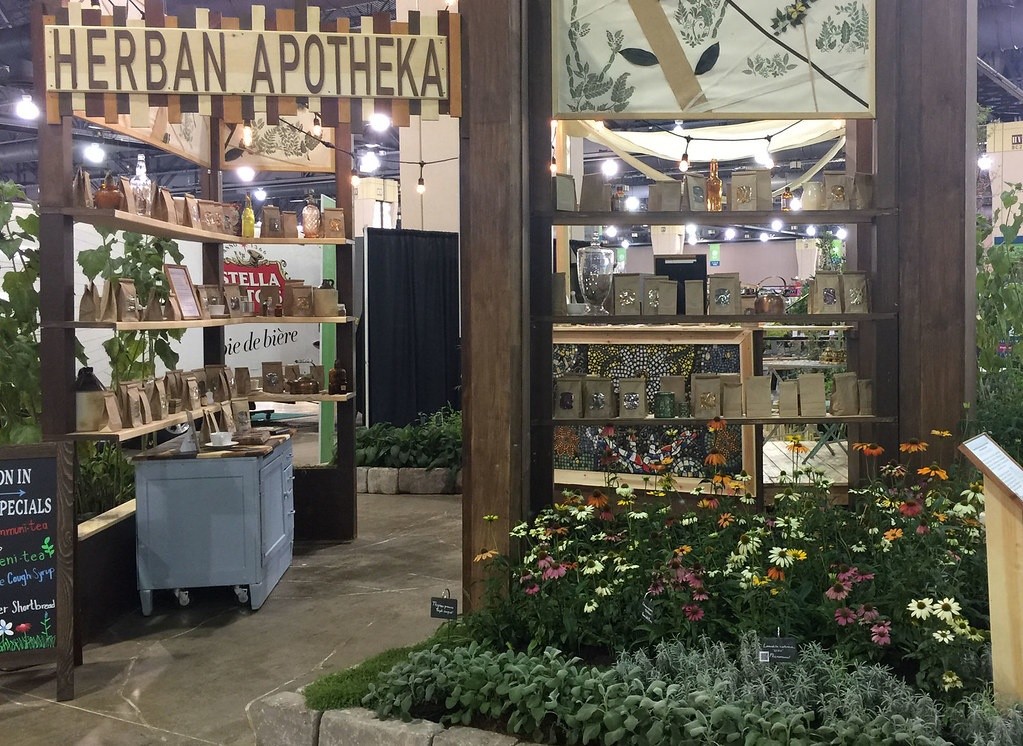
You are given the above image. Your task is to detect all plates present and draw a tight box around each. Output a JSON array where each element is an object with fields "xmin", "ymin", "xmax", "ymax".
[
  {"xmin": 205, "ymin": 441, "xmax": 239, "ymax": 450},
  {"xmin": 211, "ymin": 314, "xmax": 231, "ymax": 318}
]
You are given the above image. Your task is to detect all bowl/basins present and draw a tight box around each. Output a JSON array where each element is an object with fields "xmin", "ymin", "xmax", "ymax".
[{"xmin": 566, "ymin": 303, "xmax": 586, "ymax": 315}]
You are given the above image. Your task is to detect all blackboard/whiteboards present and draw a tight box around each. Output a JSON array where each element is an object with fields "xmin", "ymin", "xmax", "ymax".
[{"xmin": 0, "ymin": 441, "xmax": 74, "ymax": 668}]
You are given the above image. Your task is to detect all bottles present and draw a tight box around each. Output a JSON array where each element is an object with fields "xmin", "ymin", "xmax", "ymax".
[
  {"xmin": 801, "ymin": 183, "xmax": 823, "ymax": 210},
  {"xmin": 241, "ymin": 191, "xmax": 255, "ymax": 238},
  {"xmin": 576, "ymin": 232, "xmax": 614, "ymax": 316},
  {"xmin": 329, "ymin": 360, "xmax": 347, "ymax": 395},
  {"xmin": 130, "ymin": 154, "xmax": 152, "ymax": 217},
  {"xmin": 302, "ymin": 195, "xmax": 321, "ymax": 238},
  {"xmin": 781, "ymin": 186, "xmax": 795, "ymax": 211},
  {"xmin": 610, "ymin": 184, "xmax": 628, "ymax": 211},
  {"xmin": 706, "ymin": 159, "xmax": 722, "ymax": 212}
]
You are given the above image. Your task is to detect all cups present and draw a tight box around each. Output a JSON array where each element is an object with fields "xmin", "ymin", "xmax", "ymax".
[
  {"xmin": 209, "ymin": 305, "xmax": 225, "ymax": 316},
  {"xmin": 210, "ymin": 432, "xmax": 234, "ymax": 444}
]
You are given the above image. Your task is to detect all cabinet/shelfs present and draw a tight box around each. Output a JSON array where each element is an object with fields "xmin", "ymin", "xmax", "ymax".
[
  {"xmin": 32, "ymin": 0, "xmax": 359, "ymax": 646},
  {"xmin": 533, "ymin": 207, "xmax": 899, "ymax": 514}
]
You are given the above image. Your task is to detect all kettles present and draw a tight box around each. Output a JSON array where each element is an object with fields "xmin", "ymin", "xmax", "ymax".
[{"xmin": 287, "ymin": 359, "xmax": 320, "ymax": 394}]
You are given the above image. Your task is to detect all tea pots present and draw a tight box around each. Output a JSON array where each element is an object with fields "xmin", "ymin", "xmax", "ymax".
[{"xmin": 753, "ymin": 276, "xmax": 787, "ymax": 316}]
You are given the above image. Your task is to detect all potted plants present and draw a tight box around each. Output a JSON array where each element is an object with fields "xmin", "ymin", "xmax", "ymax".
[{"xmin": 356, "ymin": 402, "xmax": 463, "ymax": 495}]
